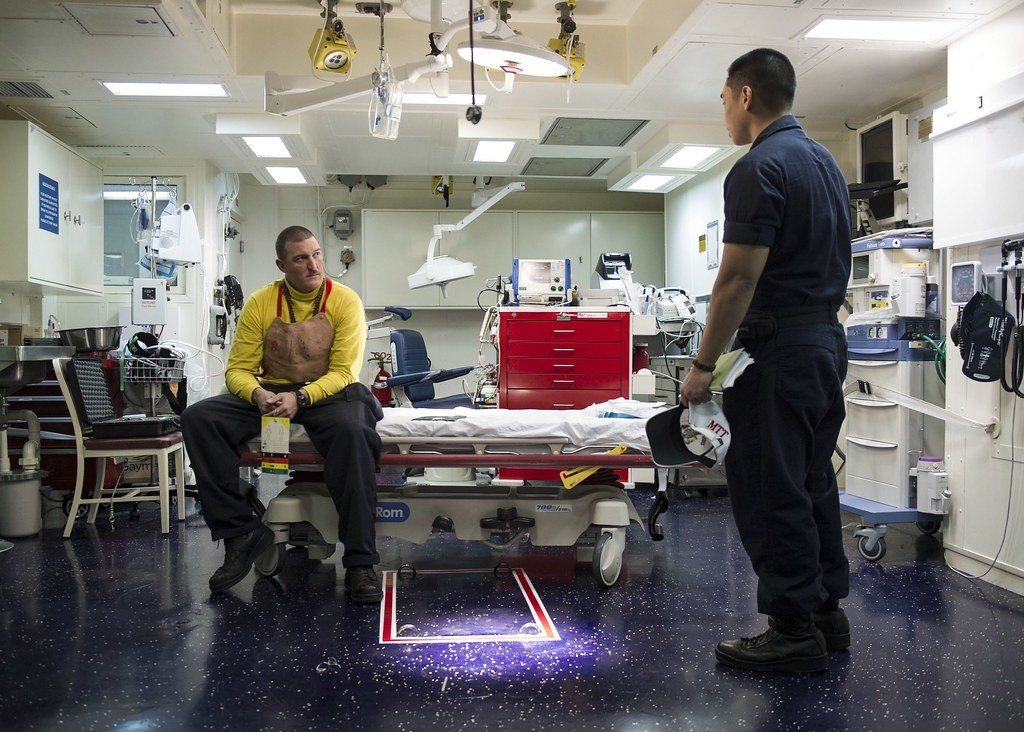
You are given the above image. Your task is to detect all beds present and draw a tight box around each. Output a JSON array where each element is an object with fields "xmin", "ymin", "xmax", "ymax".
[{"xmin": 230, "ymin": 392, "xmax": 695, "ymax": 587}]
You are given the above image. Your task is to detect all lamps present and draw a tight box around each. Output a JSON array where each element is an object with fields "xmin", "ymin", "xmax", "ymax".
[
  {"xmin": 407, "ymin": 176, "xmax": 526, "ymax": 297},
  {"xmin": 457, "ymin": 24, "xmax": 575, "ymax": 80}
]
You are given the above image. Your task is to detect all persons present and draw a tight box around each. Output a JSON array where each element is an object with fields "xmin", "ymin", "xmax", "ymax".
[
  {"xmin": 679, "ymin": 47, "xmax": 852, "ymax": 675},
  {"xmin": 180, "ymin": 226, "xmax": 384, "ymax": 604}
]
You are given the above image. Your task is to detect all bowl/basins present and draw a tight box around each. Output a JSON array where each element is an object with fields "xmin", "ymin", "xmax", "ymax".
[{"xmin": 54, "ymin": 326, "xmax": 123, "ymax": 352}]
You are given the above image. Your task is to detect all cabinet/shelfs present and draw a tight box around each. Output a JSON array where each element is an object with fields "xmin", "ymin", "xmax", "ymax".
[
  {"xmin": 358, "ymin": 207, "xmax": 664, "ymax": 313},
  {"xmin": 0, "ymin": 120, "xmax": 105, "ymax": 297},
  {"xmin": 496, "ymin": 312, "xmax": 632, "ymax": 410},
  {"xmin": 842, "ymin": 339, "xmax": 944, "ymax": 508}
]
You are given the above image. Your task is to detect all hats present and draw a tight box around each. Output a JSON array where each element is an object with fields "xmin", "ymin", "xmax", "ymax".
[{"xmin": 645, "ymin": 400, "xmax": 731, "ymax": 472}]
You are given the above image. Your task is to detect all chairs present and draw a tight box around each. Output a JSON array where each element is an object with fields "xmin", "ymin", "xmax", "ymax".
[
  {"xmin": 51, "ymin": 354, "xmax": 184, "ymax": 538},
  {"xmin": 385, "ymin": 328, "xmax": 482, "ymax": 410}
]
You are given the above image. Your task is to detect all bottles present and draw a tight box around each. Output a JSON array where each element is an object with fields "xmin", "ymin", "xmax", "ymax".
[{"xmin": 633, "ymin": 343, "xmax": 649, "ymax": 373}]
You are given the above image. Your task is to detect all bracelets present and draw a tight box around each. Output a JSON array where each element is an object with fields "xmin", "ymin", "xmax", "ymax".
[{"xmin": 691, "ymin": 360, "xmax": 717, "ymax": 373}]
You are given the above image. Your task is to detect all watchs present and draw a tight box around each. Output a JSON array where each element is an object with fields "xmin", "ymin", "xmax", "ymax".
[{"xmin": 291, "ymin": 389, "xmax": 306, "ymax": 409}]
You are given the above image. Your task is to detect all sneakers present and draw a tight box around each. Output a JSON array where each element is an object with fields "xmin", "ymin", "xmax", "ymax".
[
  {"xmin": 714, "ymin": 609, "xmax": 852, "ymax": 671},
  {"xmin": 347, "ymin": 566, "xmax": 385, "ymax": 600},
  {"xmin": 207, "ymin": 525, "xmax": 276, "ymax": 591}
]
